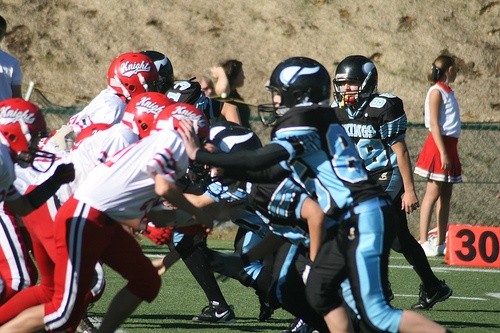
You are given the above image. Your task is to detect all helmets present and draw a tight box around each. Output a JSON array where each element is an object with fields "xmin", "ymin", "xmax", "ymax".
[
  {"xmin": 107, "ymin": 52, "xmax": 159, "ymax": 102},
  {"xmin": 151, "ymin": 103, "xmax": 209, "ymax": 145},
  {"xmin": 333, "ymin": 55, "xmax": 378, "ymax": 109},
  {"xmin": 204, "ymin": 121, "xmax": 263, "ymax": 153},
  {"xmin": 266, "ymin": 57, "xmax": 331, "ymax": 117},
  {"xmin": 121, "ymin": 92, "xmax": 171, "ymax": 139},
  {"xmin": 0, "ymin": 97, "xmax": 46, "ymax": 168},
  {"xmin": 140, "ymin": 50, "xmax": 174, "ymax": 94}
]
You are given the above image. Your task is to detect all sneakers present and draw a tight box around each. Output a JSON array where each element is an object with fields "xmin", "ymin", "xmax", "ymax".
[
  {"xmin": 192, "ymin": 304, "xmax": 237, "ymax": 323},
  {"xmin": 417, "ymin": 240, "xmax": 439, "ymax": 257},
  {"xmin": 281, "ymin": 314, "xmax": 314, "ymax": 333},
  {"xmin": 258, "ymin": 291, "xmax": 283, "ymax": 320},
  {"xmin": 412, "ymin": 279, "xmax": 453, "ymax": 310},
  {"xmin": 383, "ymin": 284, "xmax": 394, "ymax": 302},
  {"xmin": 430, "ymin": 237, "xmax": 446, "ymax": 254}
]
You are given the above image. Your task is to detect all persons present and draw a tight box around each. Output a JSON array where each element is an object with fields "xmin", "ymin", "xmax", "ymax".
[
  {"xmin": 332, "ymin": 56, "xmax": 452, "ymax": 309},
  {"xmin": 177, "ymin": 55, "xmax": 451, "ymax": 333},
  {"xmin": 0, "ymin": 104, "xmax": 213, "ymax": 333},
  {"xmin": 414, "ymin": 55, "xmax": 463, "ymax": 257},
  {"xmin": 0, "ymin": 50, "xmax": 367, "ymax": 333},
  {"xmin": 0, "ymin": 16, "xmax": 22, "ymax": 102},
  {"xmin": 0, "ymin": 98, "xmax": 75, "ymax": 305}
]
